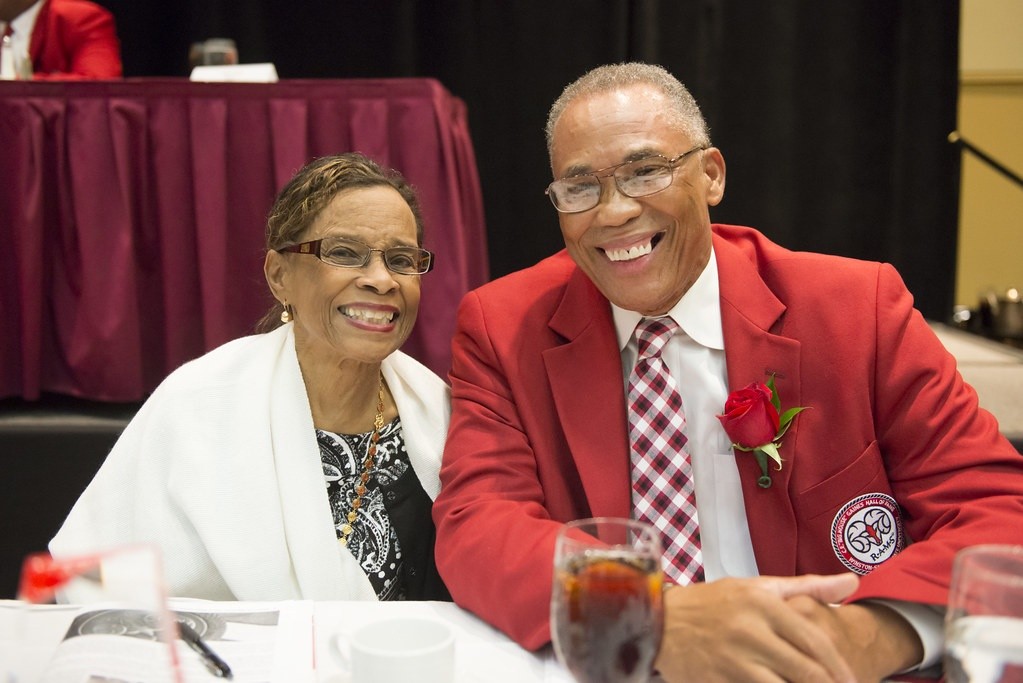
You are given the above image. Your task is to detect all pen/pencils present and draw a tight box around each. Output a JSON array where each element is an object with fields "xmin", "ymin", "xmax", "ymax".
[{"xmin": 172, "ymin": 618, "xmax": 234, "ymax": 679}]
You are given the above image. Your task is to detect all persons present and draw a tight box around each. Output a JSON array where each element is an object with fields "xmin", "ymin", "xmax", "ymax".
[
  {"xmin": 47, "ymin": 152, "xmax": 451, "ymax": 605},
  {"xmin": 0, "ymin": 0, "xmax": 122, "ymax": 82},
  {"xmin": 429, "ymin": 62, "xmax": 1023, "ymax": 683}
]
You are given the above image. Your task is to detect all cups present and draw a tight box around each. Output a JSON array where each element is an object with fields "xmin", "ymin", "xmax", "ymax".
[
  {"xmin": 330, "ymin": 615, "xmax": 454, "ymax": 683},
  {"xmin": 943, "ymin": 547, "xmax": 1023, "ymax": 683},
  {"xmin": 550, "ymin": 517, "xmax": 664, "ymax": 683}
]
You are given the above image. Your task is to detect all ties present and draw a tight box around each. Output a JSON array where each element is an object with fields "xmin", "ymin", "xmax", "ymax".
[{"xmin": 621, "ymin": 312, "xmax": 706, "ymax": 587}]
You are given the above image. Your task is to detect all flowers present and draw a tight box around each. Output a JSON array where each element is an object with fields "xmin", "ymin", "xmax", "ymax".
[{"xmin": 716, "ymin": 372, "xmax": 815, "ymax": 489}]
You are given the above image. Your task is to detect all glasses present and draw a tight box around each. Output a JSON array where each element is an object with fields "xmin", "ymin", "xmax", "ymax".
[
  {"xmin": 545, "ymin": 144, "xmax": 706, "ymax": 214},
  {"xmin": 276, "ymin": 235, "xmax": 434, "ymax": 275}
]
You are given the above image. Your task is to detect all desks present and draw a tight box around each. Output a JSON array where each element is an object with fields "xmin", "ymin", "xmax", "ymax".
[
  {"xmin": 0, "ymin": 77, "xmax": 489, "ymax": 404},
  {"xmin": 0, "ymin": 598, "xmax": 578, "ymax": 683}
]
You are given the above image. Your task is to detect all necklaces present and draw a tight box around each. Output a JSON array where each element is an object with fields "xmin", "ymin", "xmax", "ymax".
[{"xmin": 337, "ymin": 386, "xmax": 386, "ymax": 550}]
